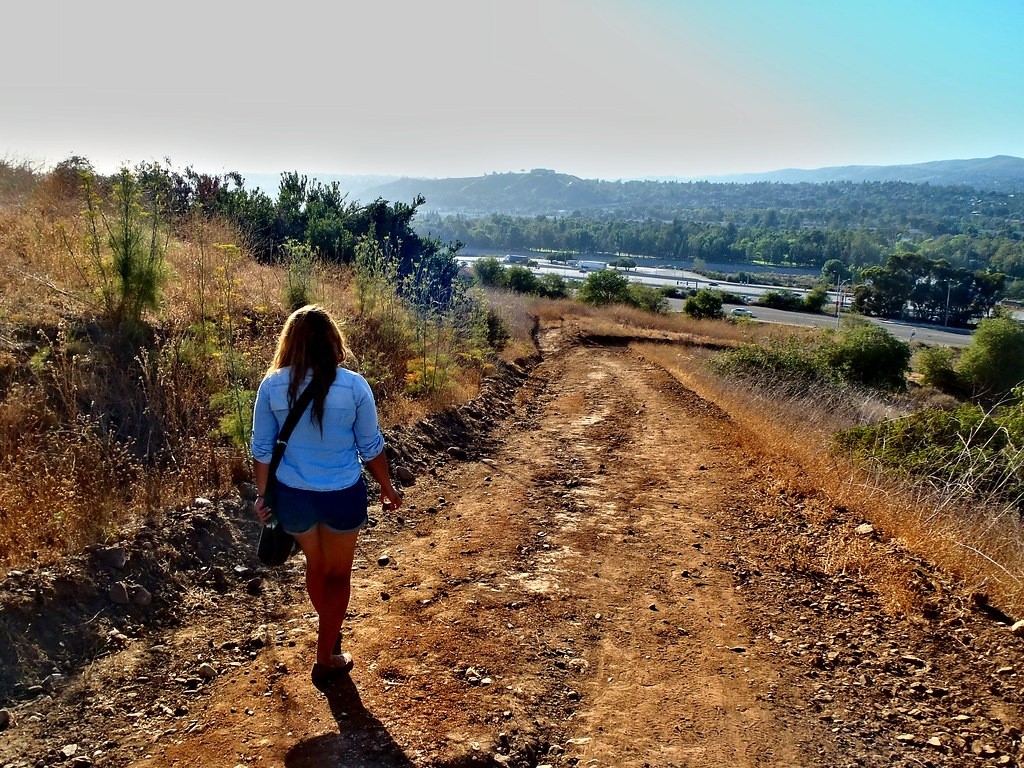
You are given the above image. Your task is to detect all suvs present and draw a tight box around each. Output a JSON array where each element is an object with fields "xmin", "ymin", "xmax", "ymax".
[{"xmin": 730, "ymin": 308, "xmax": 752, "ymax": 317}]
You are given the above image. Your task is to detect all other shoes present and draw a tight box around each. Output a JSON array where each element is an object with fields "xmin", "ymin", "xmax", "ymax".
[{"xmin": 311, "ymin": 632, "xmax": 353, "ymax": 684}]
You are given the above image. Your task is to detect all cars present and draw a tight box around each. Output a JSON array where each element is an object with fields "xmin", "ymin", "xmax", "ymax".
[
  {"xmin": 709, "ymin": 282, "xmax": 718, "ymax": 286},
  {"xmin": 579, "ymin": 269, "xmax": 587, "ymax": 273}
]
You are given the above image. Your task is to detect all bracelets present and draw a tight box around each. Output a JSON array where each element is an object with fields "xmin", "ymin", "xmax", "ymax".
[{"xmin": 256, "ymin": 494, "xmax": 265, "ymax": 498}]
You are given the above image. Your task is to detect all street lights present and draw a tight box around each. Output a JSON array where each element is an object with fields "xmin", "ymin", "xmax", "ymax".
[{"xmin": 837, "ymin": 279, "xmax": 851, "ymax": 327}]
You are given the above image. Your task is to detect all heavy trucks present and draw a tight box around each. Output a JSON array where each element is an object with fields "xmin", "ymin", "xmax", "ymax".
[
  {"xmin": 575, "ymin": 261, "xmax": 607, "ymax": 271},
  {"xmin": 503, "ymin": 255, "xmax": 528, "ymax": 264}
]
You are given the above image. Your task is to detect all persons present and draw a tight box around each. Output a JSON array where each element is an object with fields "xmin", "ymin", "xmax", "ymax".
[{"xmin": 248, "ymin": 303, "xmax": 402, "ymax": 687}]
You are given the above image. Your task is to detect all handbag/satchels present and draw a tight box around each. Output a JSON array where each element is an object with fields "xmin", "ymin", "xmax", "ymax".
[{"xmin": 257, "ymin": 512, "xmax": 295, "ymax": 567}]
